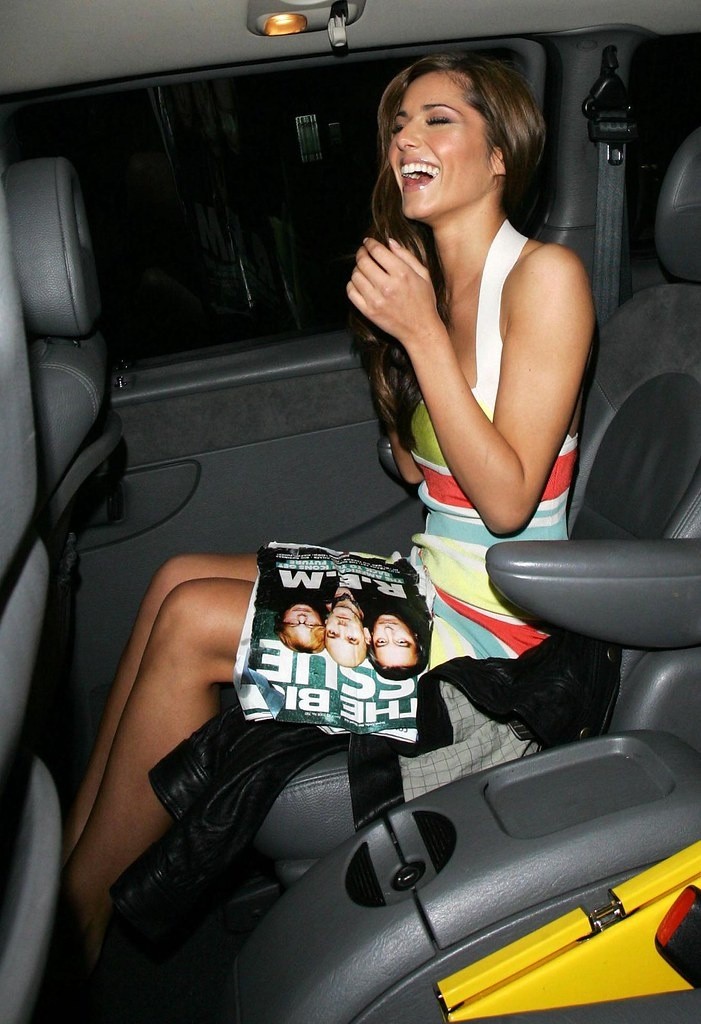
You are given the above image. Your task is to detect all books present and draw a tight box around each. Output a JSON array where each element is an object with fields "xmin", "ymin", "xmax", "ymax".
[{"xmin": 231, "ymin": 539, "xmax": 434, "ymax": 744}]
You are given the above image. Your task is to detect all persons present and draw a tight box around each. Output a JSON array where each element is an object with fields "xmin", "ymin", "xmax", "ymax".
[
  {"xmin": 255, "ymin": 546, "xmax": 431, "ymax": 680},
  {"xmin": 57, "ymin": 52, "xmax": 593, "ymax": 981}
]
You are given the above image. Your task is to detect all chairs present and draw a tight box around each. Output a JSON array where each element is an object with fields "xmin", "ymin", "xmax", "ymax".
[{"xmin": 0, "ymin": 132, "xmax": 701, "ymax": 1024}]
